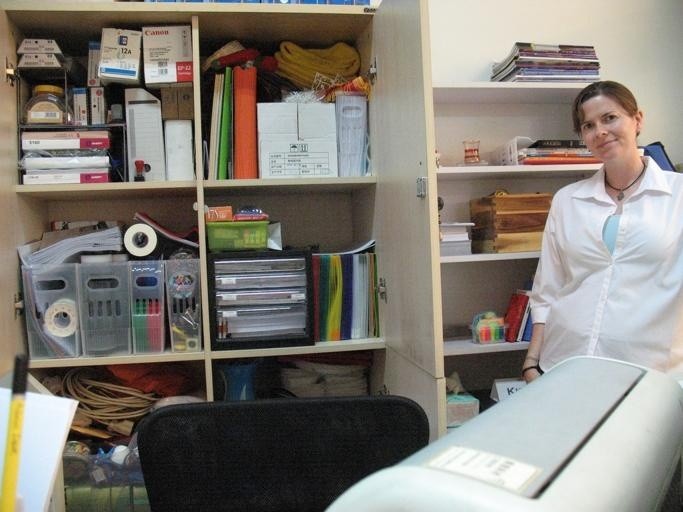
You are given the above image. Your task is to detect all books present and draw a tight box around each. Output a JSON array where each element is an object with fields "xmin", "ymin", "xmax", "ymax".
[
  {"xmin": 14, "ymin": 220, "xmax": 125, "ymax": 358},
  {"xmin": 515, "ymin": 140, "xmax": 604, "ymax": 164},
  {"xmin": 202, "ymin": 67, "xmax": 235, "ymax": 180},
  {"xmin": 490, "ymin": 42, "xmax": 601, "ymax": 84},
  {"xmin": 131, "ymin": 212, "xmax": 199, "ymax": 249}
]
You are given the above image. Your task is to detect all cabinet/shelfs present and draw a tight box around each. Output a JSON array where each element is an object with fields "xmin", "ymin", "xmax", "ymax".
[
  {"xmin": 432, "ymin": 81, "xmax": 644, "ymax": 435},
  {"xmin": 1, "ymin": 0, "xmax": 446, "ymax": 510}
]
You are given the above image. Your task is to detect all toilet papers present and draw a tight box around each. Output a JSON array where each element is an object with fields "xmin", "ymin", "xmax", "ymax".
[
  {"xmin": 164, "ymin": 119, "xmax": 195, "ymax": 180},
  {"xmin": 124, "ymin": 222, "xmax": 159, "ymax": 256},
  {"xmin": 43, "ymin": 298, "xmax": 78, "ymax": 339}
]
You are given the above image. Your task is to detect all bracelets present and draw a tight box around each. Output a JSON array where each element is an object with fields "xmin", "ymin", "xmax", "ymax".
[{"xmin": 521, "ymin": 365, "xmax": 537, "ymax": 377}]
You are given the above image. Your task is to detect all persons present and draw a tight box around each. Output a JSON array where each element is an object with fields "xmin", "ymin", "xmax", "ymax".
[{"xmin": 522, "ymin": 81, "xmax": 682, "ymax": 390}]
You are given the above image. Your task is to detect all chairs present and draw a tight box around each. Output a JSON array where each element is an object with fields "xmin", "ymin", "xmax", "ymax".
[{"xmin": 137, "ymin": 394, "xmax": 431, "ymax": 512}]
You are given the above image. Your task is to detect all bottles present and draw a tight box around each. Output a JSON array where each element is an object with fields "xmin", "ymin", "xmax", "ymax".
[{"xmin": 20, "ymin": 83, "xmax": 75, "ymax": 125}]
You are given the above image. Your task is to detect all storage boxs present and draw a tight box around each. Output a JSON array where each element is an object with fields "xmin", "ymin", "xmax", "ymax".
[
  {"xmin": 98, "ymin": 25, "xmax": 338, "ymax": 179},
  {"xmin": 205, "ymin": 221, "xmax": 270, "ymax": 251}
]
